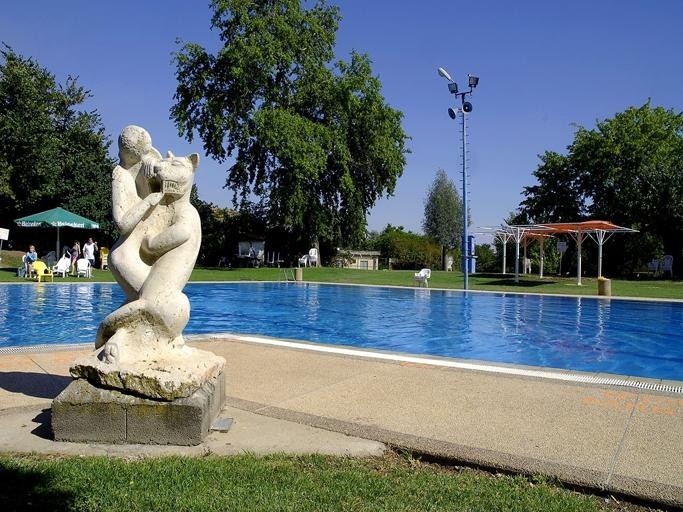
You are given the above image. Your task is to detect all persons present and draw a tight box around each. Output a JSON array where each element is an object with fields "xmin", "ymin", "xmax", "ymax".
[
  {"xmin": 102, "ymin": 123, "xmax": 160, "ymax": 301},
  {"xmin": 68, "ymin": 239, "xmax": 81, "ymax": 276},
  {"xmin": 217, "ymin": 255, "xmax": 228, "ymax": 267},
  {"xmin": 6, "ymin": 238, "xmax": 14, "ymax": 249},
  {"xmin": 81, "ymin": 237, "xmax": 98, "ymax": 278},
  {"xmin": 23, "ymin": 244, "xmax": 37, "ymax": 278},
  {"xmin": 308, "ymin": 244, "xmax": 317, "ymax": 257},
  {"xmin": 224, "ymin": 255, "xmax": 240, "ymax": 269},
  {"xmin": 254, "ymin": 246, "xmax": 265, "ymax": 268}
]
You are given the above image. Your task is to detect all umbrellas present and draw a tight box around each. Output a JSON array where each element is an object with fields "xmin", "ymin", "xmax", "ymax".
[{"xmin": 13, "ymin": 206, "xmax": 100, "ymax": 262}]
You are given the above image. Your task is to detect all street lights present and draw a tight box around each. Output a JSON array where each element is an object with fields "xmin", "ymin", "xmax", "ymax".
[{"xmin": 435, "ymin": 63, "xmax": 484, "ymax": 297}]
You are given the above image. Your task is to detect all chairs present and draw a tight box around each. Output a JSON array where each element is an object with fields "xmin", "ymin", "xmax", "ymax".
[
  {"xmin": 647, "ymin": 255, "xmax": 673, "ymax": 279},
  {"xmin": 414, "ymin": 268, "xmax": 431, "ymax": 288},
  {"xmin": 298, "ymin": 248, "xmax": 318, "ymax": 268},
  {"xmin": 22, "ymin": 246, "xmax": 111, "ymax": 283}
]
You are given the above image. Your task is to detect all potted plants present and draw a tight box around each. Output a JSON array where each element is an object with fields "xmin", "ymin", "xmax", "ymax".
[{"xmin": 597, "ymin": 276, "xmax": 611, "ymax": 295}]
